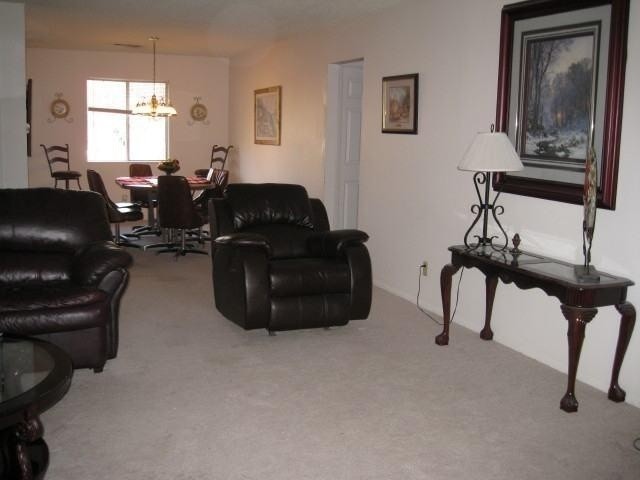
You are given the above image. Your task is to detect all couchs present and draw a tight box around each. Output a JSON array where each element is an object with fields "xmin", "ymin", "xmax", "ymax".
[
  {"xmin": 0, "ymin": 186, "xmax": 135, "ymax": 374},
  {"xmin": 208, "ymin": 183, "xmax": 374, "ymax": 336}
]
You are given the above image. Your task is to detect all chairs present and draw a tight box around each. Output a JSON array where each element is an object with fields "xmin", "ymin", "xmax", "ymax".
[{"xmin": 38, "ymin": 140, "xmax": 234, "ymax": 258}]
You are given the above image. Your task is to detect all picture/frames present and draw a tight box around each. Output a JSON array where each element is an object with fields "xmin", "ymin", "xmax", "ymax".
[
  {"xmin": 253, "ymin": 86, "xmax": 283, "ymax": 146},
  {"xmin": 492, "ymin": 1, "xmax": 629, "ymax": 212},
  {"xmin": 381, "ymin": 72, "xmax": 419, "ymax": 136}
]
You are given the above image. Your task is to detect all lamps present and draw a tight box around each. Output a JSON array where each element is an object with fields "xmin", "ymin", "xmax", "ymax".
[
  {"xmin": 130, "ymin": 36, "xmax": 179, "ymax": 119},
  {"xmin": 456, "ymin": 122, "xmax": 524, "ymax": 250}
]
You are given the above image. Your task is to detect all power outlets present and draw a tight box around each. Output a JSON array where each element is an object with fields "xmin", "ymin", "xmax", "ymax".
[{"xmin": 422, "ymin": 262, "xmax": 429, "ymax": 278}]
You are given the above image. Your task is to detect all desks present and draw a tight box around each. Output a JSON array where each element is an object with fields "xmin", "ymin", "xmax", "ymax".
[{"xmin": 432, "ymin": 242, "xmax": 636, "ymax": 415}]
